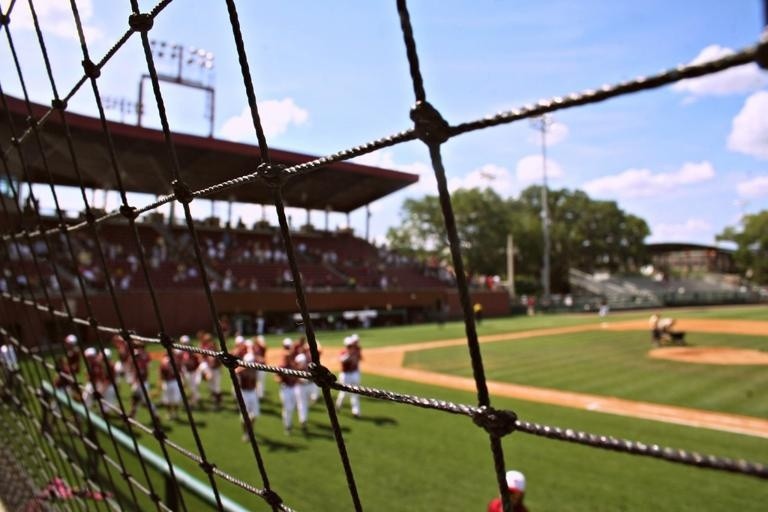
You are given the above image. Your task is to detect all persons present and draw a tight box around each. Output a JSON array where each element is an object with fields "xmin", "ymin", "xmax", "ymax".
[
  {"xmin": 474, "ymin": 301, "xmax": 482, "ymax": 322},
  {"xmin": 53, "ymin": 334, "xmax": 363, "ymax": 440},
  {"xmin": 598, "ymin": 297, "xmax": 608, "ymax": 317},
  {"xmin": 519, "ymin": 294, "xmax": 553, "ymax": 316},
  {"xmin": 564, "ymin": 294, "xmax": 573, "ymax": 313},
  {"xmin": 649, "ymin": 312, "xmax": 674, "ymax": 348},
  {"xmin": 219, "ymin": 307, "xmax": 266, "ymax": 338},
  {"xmin": 486, "ymin": 469, "xmax": 530, "ymax": 511},
  {"xmin": 2, "ymin": 229, "xmax": 501, "ymax": 292}
]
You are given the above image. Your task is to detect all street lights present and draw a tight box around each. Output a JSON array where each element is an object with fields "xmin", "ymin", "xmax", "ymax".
[
  {"xmin": 127, "ymin": 39, "xmax": 218, "ymax": 141},
  {"xmin": 100, "ymin": 97, "xmax": 145, "ymax": 127},
  {"xmin": 531, "ymin": 112, "xmax": 556, "ymax": 306}
]
